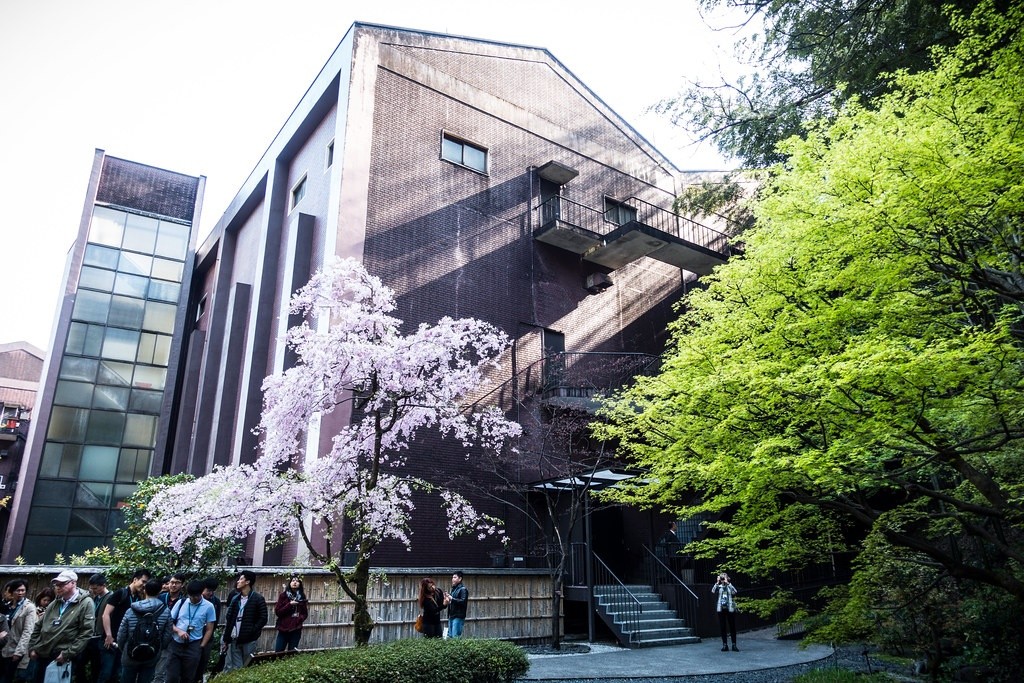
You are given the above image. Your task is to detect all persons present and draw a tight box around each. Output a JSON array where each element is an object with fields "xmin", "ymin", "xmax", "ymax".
[
  {"xmin": 26, "ymin": 569, "xmax": 96, "ymax": 683},
  {"xmin": 97, "ymin": 569, "xmax": 152, "ymax": 683},
  {"xmin": 663, "ymin": 521, "xmax": 684, "ymax": 584},
  {"xmin": 711, "ymin": 572, "xmax": 739, "ymax": 652},
  {"xmin": 419, "ymin": 578, "xmax": 451, "ymax": 639},
  {"xmin": 273, "ymin": 574, "xmax": 309, "ymax": 653},
  {"xmin": 116, "ymin": 579, "xmax": 173, "ymax": 683},
  {"xmin": 0, "ymin": 569, "xmax": 267, "ymax": 683},
  {"xmin": 165, "ymin": 580, "xmax": 217, "ymax": 683},
  {"xmin": 443, "ymin": 570, "xmax": 469, "ymax": 639}
]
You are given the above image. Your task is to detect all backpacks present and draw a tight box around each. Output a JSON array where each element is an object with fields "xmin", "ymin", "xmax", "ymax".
[{"xmin": 127, "ymin": 603, "xmax": 167, "ymax": 662}]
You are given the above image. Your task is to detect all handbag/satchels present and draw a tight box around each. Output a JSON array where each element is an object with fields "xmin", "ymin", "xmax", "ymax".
[
  {"xmin": 207, "ymin": 651, "xmax": 227, "ymax": 682},
  {"xmin": 44, "ymin": 659, "xmax": 71, "ymax": 683},
  {"xmin": 415, "ymin": 615, "xmax": 424, "ymax": 633}
]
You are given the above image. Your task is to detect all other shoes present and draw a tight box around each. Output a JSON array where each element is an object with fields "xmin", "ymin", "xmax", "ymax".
[
  {"xmin": 721, "ymin": 646, "xmax": 730, "ymax": 652},
  {"xmin": 732, "ymin": 645, "xmax": 739, "ymax": 652}
]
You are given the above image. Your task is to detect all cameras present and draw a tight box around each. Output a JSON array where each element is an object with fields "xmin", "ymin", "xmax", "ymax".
[{"xmin": 720, "ymin": 576, "xmax": 725, "ymax": 580}]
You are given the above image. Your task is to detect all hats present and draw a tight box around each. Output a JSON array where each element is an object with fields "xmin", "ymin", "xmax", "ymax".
[{"xmin": 51, "ymin": 570, "xmax": 77, "ymax": 583}]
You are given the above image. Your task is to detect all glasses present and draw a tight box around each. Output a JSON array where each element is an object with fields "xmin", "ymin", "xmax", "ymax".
[
  {"xmin": 170, "ymin": 581, "xmax": 184, "ymax": 585},
  {"xmin": 53, "ymin": 580, "xmax": 73, "ymax": 587}
]
